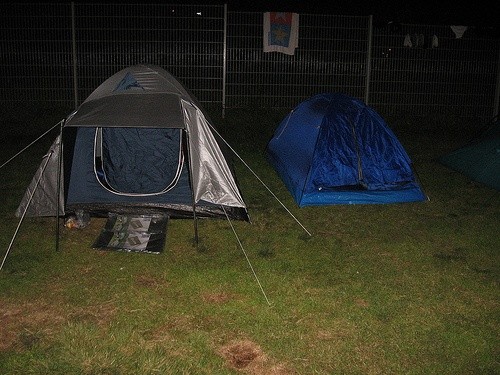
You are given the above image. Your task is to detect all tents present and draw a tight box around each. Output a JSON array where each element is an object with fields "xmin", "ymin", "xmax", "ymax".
[
  {"xmin": 14, "ymin": 63, "xmax": 253, "ymax": 254},
  {"xmin": 262, "ymin": 93, "xmax": 430, "ymax": 208}
]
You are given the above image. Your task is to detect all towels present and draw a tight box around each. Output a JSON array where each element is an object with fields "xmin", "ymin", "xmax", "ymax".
[{"xmin": 262, "ymin": 10, "xmax": 299, "ymax": 56}]
